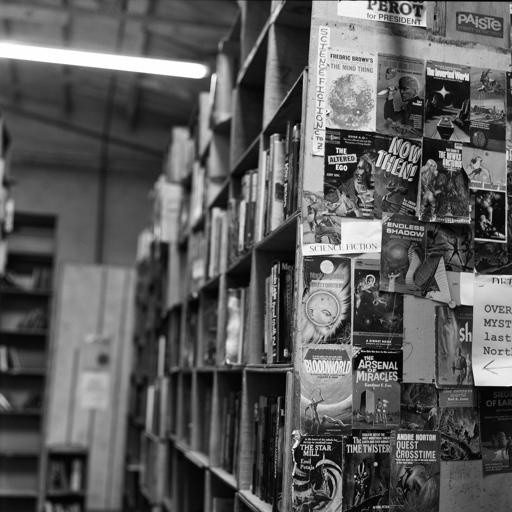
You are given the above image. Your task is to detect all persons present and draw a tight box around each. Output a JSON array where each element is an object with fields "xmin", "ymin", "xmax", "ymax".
[
  {"xmin": 356, "ymin": 158, "xmax": 374, "ymax": 190},
  {"xmin": 452, "ymin": 348, "xmax": 467, "ymax": 385},
  {"xmin": 304, "ymin": 389, "xmax": 324, "ymax": 434},
  {"xmin": 446, "ymin": 236, "xmax": 464, "ymax": 272},
  {"xmin": 421, "ymin": 159, "xmax": 437, "ymax": 221},
  {"xmin": 382, "ymin": 400, "xmax": 389, "ymax": 423},
  {"xmin": 462, "ymin": 232, "xmax": 473, "ymax": 270},
  {"xmin": 322, "ymin": 415, "xmax": 345, "ymax": 428},
  {"xmin": 384, "ymin": 76, "xmax": 423, "ymax": 138},
  {"xmin": 375, "ymin": 398, "xmax": 383, "ymax": 423}
]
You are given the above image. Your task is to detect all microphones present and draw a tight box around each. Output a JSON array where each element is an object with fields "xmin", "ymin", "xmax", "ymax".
[{"xmin": 43, "ymin": 444, "xmax": 89, "ymax": 512}]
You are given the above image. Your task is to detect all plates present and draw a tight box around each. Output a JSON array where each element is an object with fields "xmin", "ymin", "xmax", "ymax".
[
  {"xmin": 0, "ymin": 210, "xmax": 59, "ymax": 512},
  {"xmin": 122, "ymin": 1, "xmax": 511, "ymax": 511}
]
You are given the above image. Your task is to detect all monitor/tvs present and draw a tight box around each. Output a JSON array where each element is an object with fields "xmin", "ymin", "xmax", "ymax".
[
  {"xmin": 203, "ymin": 122, "xmax": 300, "ymax": 512},
  {"xmin": 0, "ymin": 256, "xmax": 86, "ymax": 512}
]
